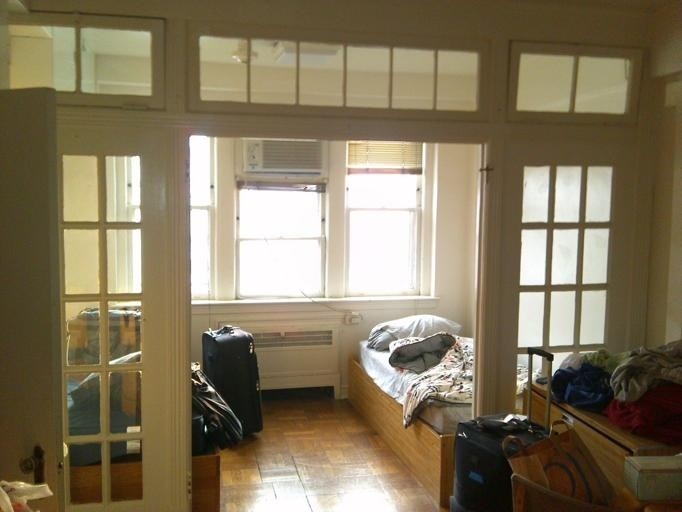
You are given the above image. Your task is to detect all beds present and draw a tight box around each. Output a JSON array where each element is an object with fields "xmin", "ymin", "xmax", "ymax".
[{"xmin": 350, "ymin": 334, "xmax": 524, "ymax": 509}]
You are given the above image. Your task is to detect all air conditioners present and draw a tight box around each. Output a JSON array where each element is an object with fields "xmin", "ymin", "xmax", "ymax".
[{"xmin": 243, "ymin": 137, "xmax": 325, "ymax": 174}]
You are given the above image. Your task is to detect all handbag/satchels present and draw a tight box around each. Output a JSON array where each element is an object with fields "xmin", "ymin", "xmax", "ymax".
[{"xmin": 502, "ymin": 418, "xmax": 618, "ymax": 505}]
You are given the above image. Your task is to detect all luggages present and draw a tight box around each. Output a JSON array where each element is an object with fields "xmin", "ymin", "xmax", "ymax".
[
  {"xmin": 450, "ymin": 348, "xmax": 554, "ymax": 512},
  {"xmin": 203, "ymin": 324, "xmax": 264, "ymax": 437}
]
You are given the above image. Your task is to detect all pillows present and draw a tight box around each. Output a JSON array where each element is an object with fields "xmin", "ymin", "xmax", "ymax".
[{"xmin": 369, "ymin": 314, "xmax": 463, "ymax": 351}]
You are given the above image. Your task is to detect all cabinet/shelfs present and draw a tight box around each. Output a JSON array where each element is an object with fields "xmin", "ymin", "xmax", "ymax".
[{"xmin": 523, "ymin": 381, "xmax": 672, "ymax": 459}]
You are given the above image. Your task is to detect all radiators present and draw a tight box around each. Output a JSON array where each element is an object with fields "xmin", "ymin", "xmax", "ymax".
[{"xmin": 223, "ymin": 323, "xmax": 342, "ymax": 398}]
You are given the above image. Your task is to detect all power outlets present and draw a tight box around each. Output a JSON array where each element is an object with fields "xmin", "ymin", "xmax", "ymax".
[{"xmin": 345, "ymin": 313, "xmax": 362, "ymax": 326}]
